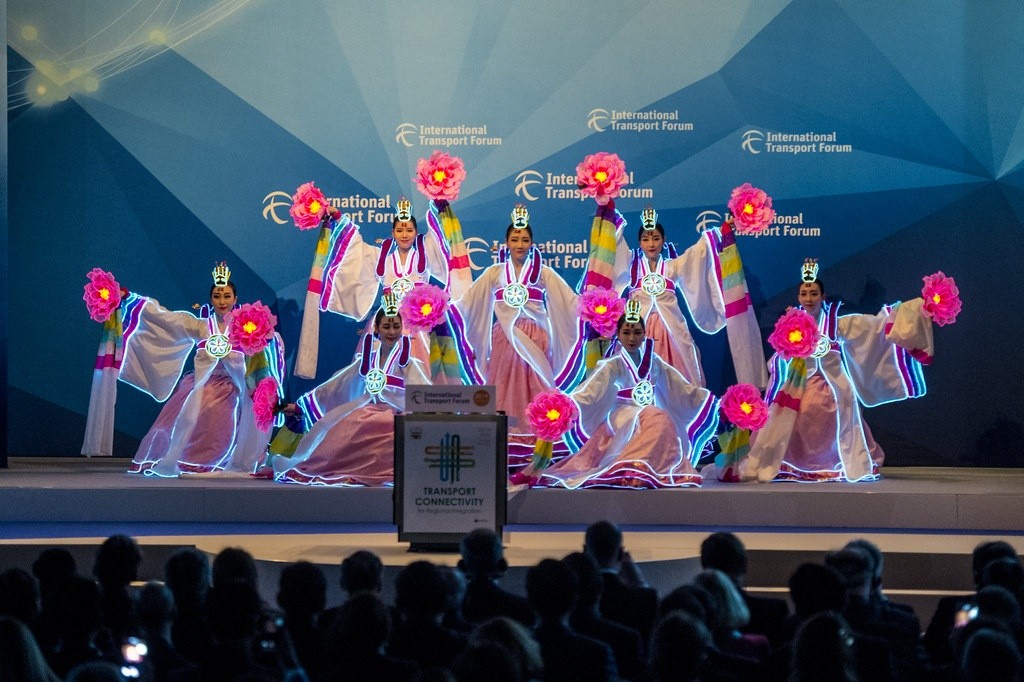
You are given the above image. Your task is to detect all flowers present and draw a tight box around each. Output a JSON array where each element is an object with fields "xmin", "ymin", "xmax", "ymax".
[
  {"xmin": 574, "ymin": 152, "xmax": 630, "ymax": 206},
  {"xmin": 412, "ymin": 151, "xmax": 465, "ymax": 200},
  {"xmin": 576, "ymin": 286, "xmax": 626, "ymax": 340},
  {"xmin": 921, "ymin": 270, "xmax": 963, "ymax": 326},
  {"xmin": 223, "ymin": 301, "xmax": 276, "ymax": 355},
  {"xmin": 768, "ymin": 306, "xmax": 821, "ymax": 359},
  {"xmin": 526, "ymin": 387, "xmax": 579, "ymax": 442},
  {"xmin": 727, "ymin": 182, "xmax": 775, "ymax": 232},
  {"xmin": 82, "ymin": 268, "xmax": 121, "ymax": 323},
  {"xmin": 720, "ymin": 381, "xmax": 770, "ymax": 431},
  {"xmin": 252, "ymin": 376, "xmax": 287, "ymax": 433},
  {"xmin": 290, "ymin": 180, "xmax": 330, "ymax": 231},
  {"xmin": 398, "ymin": 284, "xmax": 451, "ymax": 333}
]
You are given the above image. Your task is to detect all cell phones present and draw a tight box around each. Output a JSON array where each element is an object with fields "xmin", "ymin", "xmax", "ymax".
[
  {"xmin": 122, "ymin": 636, "xmax": 151, "ymax": 682},
  {"xmin": 258, "ymin": 607, "xmax": 286, "ymax": 653}
]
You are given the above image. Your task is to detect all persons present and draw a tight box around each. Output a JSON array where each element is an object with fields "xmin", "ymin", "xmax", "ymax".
[
  {"xmin": 82, "ymin": 195, "xmax": 933, "ymax": 489},
  {"xmin": 1, "ymin": 520, "xmax": 1024, "ymax": 682}
]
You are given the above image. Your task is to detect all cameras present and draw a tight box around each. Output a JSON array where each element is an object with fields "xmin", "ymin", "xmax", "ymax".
[{"xmin": 954, "ymin": 602, "xmax": 979, "ymax": 628}]
